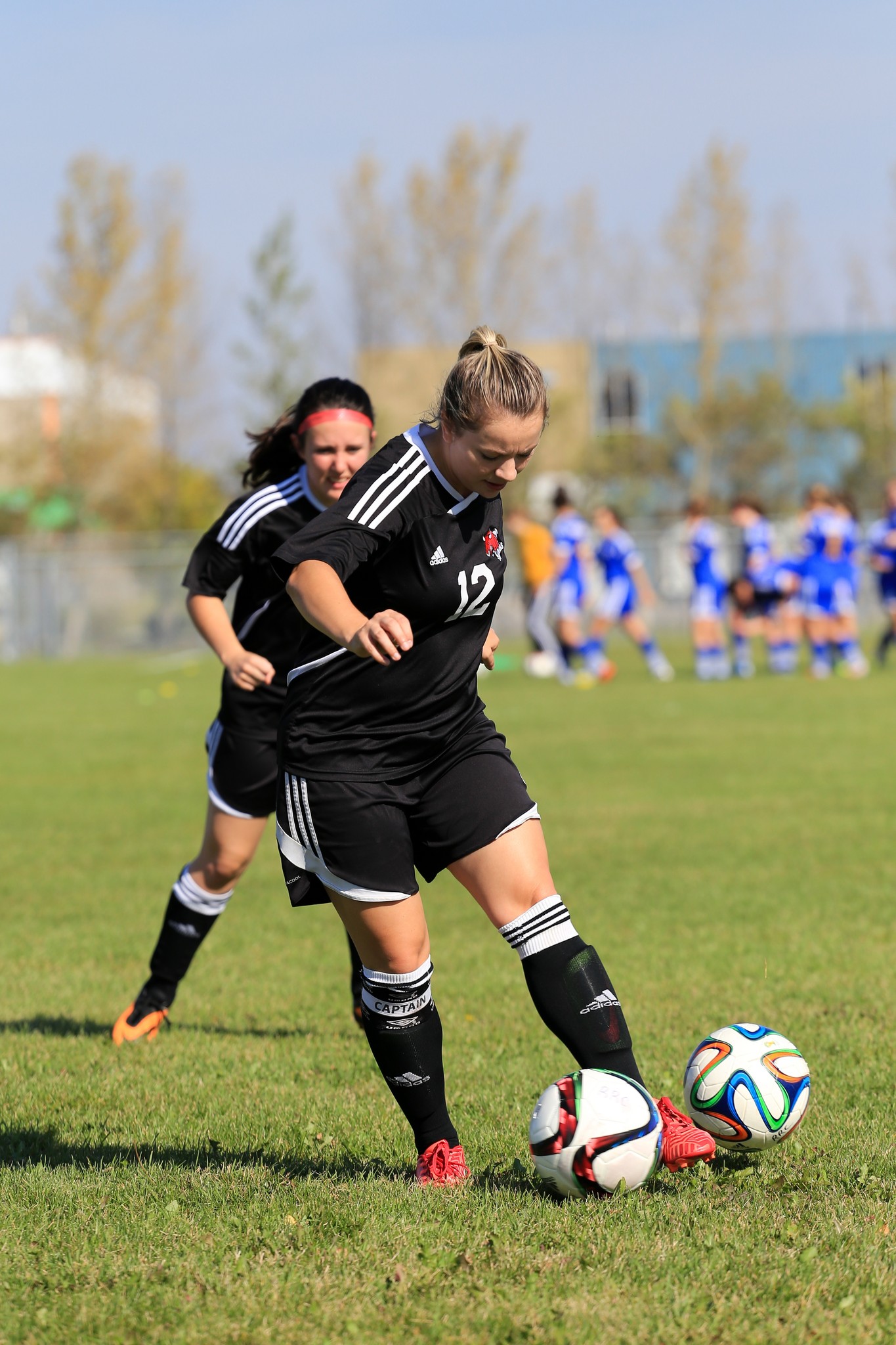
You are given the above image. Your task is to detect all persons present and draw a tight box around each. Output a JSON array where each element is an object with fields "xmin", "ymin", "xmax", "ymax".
[
  {"xmin": 110, "ymin": 376, "xmax": 380, "ymax": 1044},
  {"xmin": 271, "ymin": 324, "xmax": 715, "ymax": 1187},
  {"xmin": 503, "ymin": 485, "xmax": 896, "ymax": 690}
]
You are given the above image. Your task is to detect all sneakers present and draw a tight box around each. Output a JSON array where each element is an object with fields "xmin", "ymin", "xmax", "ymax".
[
  {"xmin": 414, "ymin": 1139, "xmax": 466, "ymax": 1190},
  {"xmin": 112, "ymin": 978, "xmax": 174, "ymax": 1049},
  {"xmin": 655, "ymin": 1095, "xmax": 716, "ymax": 1177}
]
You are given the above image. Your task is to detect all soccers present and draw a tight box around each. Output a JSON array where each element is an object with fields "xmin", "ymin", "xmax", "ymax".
[
  {"xmin": 685, "ymin": 1021, "xmax": 812, "ymax": 1154},
  {"xmin": 526, "ymin": 1067, "xmax": 663, "ymax": 1198}
]
